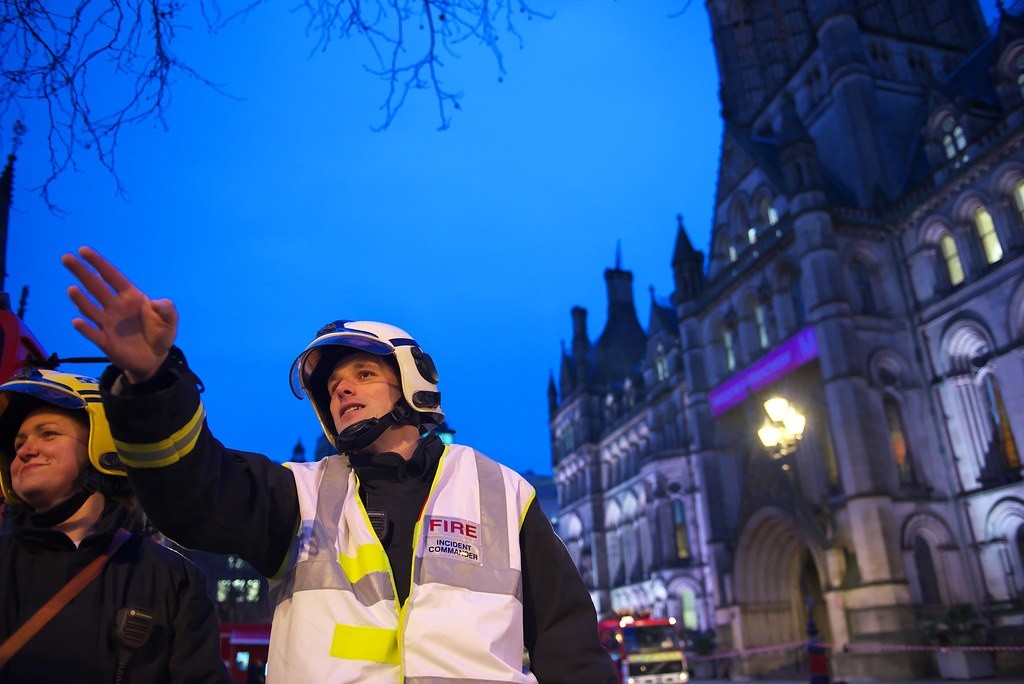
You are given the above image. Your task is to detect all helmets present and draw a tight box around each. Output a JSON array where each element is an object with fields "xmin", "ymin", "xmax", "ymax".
[
  {"xmin": 288, "ymin": 316, "xmax": 456, "ymax": 455},
  {"xmin": 0, "ymin": 367, "xmax": 128, "ymax": 516}
]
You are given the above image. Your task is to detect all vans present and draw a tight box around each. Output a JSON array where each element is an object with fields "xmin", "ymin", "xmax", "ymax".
[{"xmin": 597, "ymin": 618, "xmax": 690, "ymax": 684}]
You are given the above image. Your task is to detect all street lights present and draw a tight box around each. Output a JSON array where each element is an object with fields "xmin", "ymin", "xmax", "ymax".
[{"xmin": 759, "ymin": 398, "xmax": 830, "ymax": 683}]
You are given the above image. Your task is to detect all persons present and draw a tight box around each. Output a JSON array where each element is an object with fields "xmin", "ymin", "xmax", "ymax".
[
  {"xmin": 62, "ymin": 247, "xmax": 623, "ymax": 684},
  {"xmin": 0, "ymin": 369, "xmax": 221, "ymax": 684}
]
